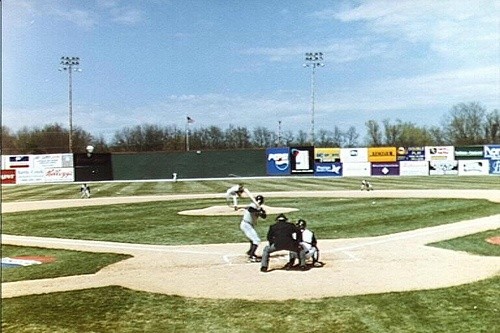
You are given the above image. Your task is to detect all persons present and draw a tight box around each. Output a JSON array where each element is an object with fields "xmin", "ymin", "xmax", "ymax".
[
  {"xmin": 259, "ymin": 213, "xmax": 309, "ymax": 271},
  {"xmin": 227, "ymin": 183, "xmax": 244, "ymax": 211},
  {"xmin": 81, "ymin": 184, "xmax": 91, "ymax": 199},
  {"xmin": 285, "ymin": 220, "xmax": 322, "ymax": 268},
  {"xmin": 360, "ymin": 179, "xmax": 374, "ymax": 191},
  {"xmin": 239, "ymin": 196, "xmax": 266, "ymax": 262}
]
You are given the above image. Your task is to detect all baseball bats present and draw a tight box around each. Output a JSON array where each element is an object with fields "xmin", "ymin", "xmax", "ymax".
[{"xmin": 243, "ymin": 188, "xmax": 263, "ymax": 214}]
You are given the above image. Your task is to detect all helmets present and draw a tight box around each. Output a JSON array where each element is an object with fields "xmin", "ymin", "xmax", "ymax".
[
  {"xmin": 296, "ymin": 220, "xmax": 306, "ymax": 227},
  {"xmin": 256, "ymin": 195, "xmax": 263, "ymax": 203}
]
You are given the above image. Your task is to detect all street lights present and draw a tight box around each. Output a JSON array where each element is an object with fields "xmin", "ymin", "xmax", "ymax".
[
  {"xmin": 57, "ymin": 55, "xmax": 82, "ymax": 153},
  {"xmin": 302, "ymin": 51, "xmax": 328, "ymax": 147}
]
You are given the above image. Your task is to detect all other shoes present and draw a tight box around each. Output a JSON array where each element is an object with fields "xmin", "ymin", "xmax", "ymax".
[
  {"xmin": 300, "ymin": 266, "xmax": 309, "ymax": 270},
  {"xmin": 261, "ymin": 267, "xmax": 267, "ymax": 271},
  {"xmin": 250, "ymin": 255, "xmax": 261, "ymax": 262},
  {"xmin": 313, "ymin": 262, "xmax": 322, "ymax": 267}
]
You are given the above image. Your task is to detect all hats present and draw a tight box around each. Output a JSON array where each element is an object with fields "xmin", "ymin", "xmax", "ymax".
[{"xmin": 276, "ymin": 213, "xmax": 288, "ymax": 220}]
